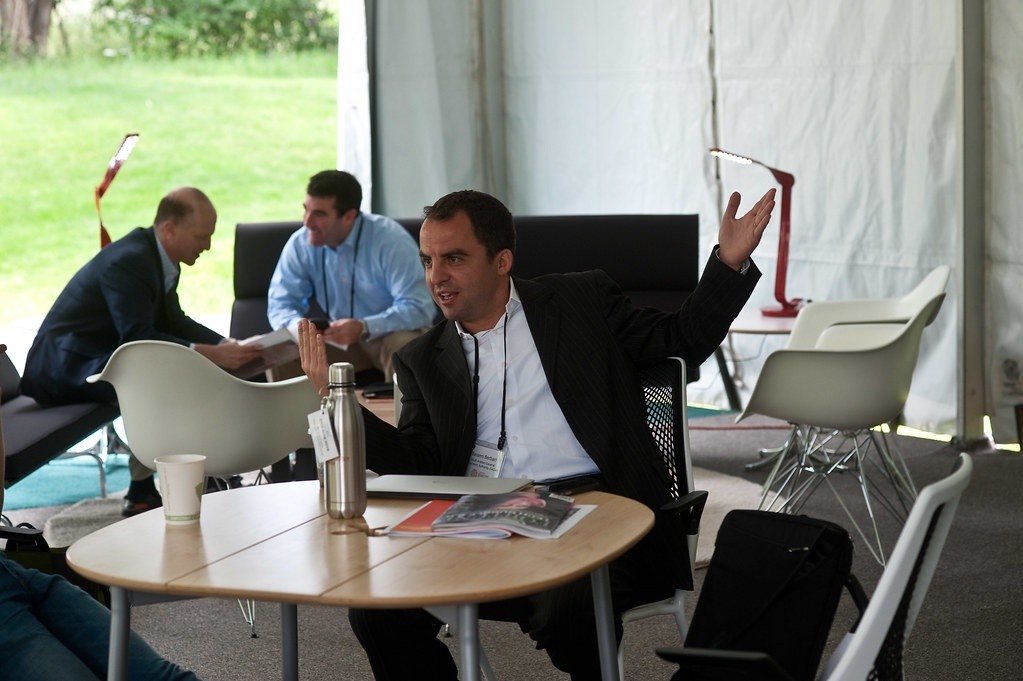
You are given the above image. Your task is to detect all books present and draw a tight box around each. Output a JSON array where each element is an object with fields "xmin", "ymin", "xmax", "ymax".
[
  {"xmin": 387, "ymin": 490, "xmax": 599, "ymax": 540},
  {"xmin": 225, "ymin": 327, "xmax": 301, "ymax": 380}
]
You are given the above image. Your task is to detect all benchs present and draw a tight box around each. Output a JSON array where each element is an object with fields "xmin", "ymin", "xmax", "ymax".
[
  {"xmin": 0, "ymin": 344, "xmax": 121, "ymax": 537},
  {"xmin": 228, "ymin": 213, "xmax": 701, "ymax": 386}
]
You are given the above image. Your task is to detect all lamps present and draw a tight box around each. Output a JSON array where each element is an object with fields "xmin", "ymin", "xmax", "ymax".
[{"xmin": 710, "ymin": 146, "xmax": 805, "ymax": 318}]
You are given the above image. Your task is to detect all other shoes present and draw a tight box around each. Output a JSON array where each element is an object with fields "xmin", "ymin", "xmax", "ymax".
[{"xmin": 122, "ymin": 495, "xmax": 160, "ymax": 518}]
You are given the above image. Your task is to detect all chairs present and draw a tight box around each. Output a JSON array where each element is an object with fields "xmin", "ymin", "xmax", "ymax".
[
  {"xmin": 84, "ymin": 339, "xmax": 321, "ymax": 642},
  {"xmin": 744, "ymin": 261, "xmax": 954, "ymax": 515},
  {"xmin": 655, "ymin": 455, "xmax": 976, "ymax": 681},
  {"xmin": 734, "ymin": 292, "xmax": 948, "ymax": 569},
  {"xmin": 394, "ymin": 353, "xmax": 711, "ymax": 681}
]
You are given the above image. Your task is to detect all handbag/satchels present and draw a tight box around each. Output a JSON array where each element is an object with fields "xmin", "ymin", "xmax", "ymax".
[{"xmin": 669, "ymin": 510, "xmax": 854, "ymax": 681}]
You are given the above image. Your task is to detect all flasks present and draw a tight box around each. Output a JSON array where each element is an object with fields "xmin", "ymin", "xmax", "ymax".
[{"xmin": 319, "ymin": 362, "xmax": 367, "ymax": 520}]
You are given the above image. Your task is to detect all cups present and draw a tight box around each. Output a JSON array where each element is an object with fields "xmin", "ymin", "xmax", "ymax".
[{"xmin": 154, "ymin": 454, "xmax": 207, "ymax": 526}]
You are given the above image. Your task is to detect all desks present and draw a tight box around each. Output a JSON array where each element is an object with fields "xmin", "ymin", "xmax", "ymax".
[
  {"xmin": 726, "ymin": 304, "xmax": 797, "ymax": 335},
  {"xmin": 64, "ymin": 477, "xmax": 655, "ymax": 681},
  {"xmin": 353, "ymin": 388, "xmax": 397, "ymax": 430}
]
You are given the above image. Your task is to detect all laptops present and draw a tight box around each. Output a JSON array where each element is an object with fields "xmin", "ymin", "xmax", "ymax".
[{"xmin": 364, "ymin": 474, "xmax": 535, "ymax": 500}]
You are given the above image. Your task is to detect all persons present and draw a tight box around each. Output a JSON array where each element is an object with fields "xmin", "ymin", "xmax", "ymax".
[
  {"xmin": 0, "ymin": 411, "xmax": 203, "ymax": 681},
  {"xmin": 297, "ymin": 189, "xmax": 775, "ymax": 681},
  {"xmin": 263, "ymin": 168, "xmax": 439, "ymax": 484},
  {"xmin": 21, "ymin": 187, "xmax": 274, "ymax": 517}
]
